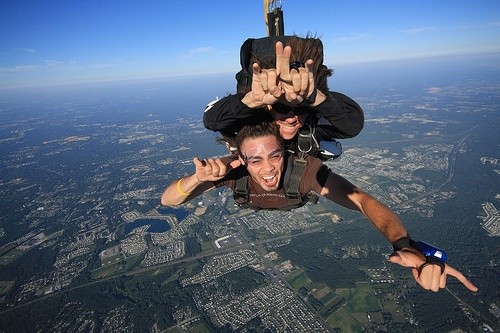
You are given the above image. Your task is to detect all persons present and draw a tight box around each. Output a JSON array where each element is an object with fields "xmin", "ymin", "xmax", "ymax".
[
  {"xmin": 202, "ymin": 40, "xmax": 364, "ymax": 148},
  {"xmin": 160, "ymin": 115, "xmax": 482, "ymax": 296}
]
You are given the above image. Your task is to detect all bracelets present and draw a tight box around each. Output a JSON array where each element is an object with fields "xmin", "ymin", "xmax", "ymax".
[{"xmin": 176, "ymin": 177, "xmax": 191, "ymax": 197}]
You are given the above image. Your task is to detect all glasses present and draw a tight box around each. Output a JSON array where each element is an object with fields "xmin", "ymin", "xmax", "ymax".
[{"xmin": 271, "ymin": 102, "xmax": 310, "ymax": 116}]
